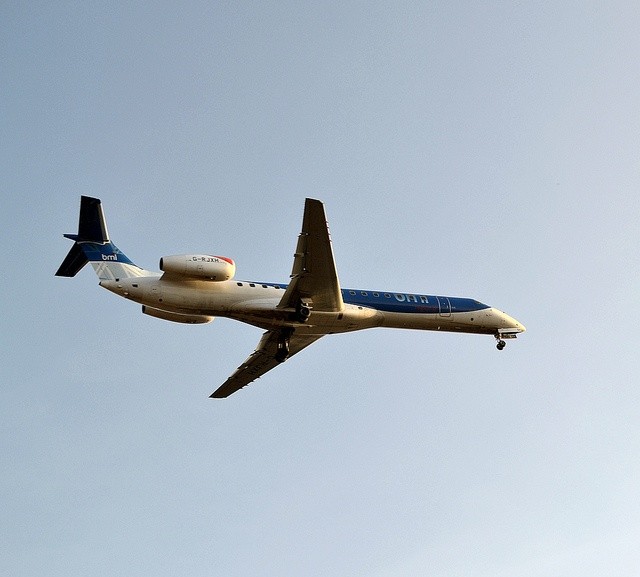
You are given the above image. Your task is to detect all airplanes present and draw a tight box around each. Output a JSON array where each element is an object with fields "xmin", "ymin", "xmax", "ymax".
[{"xmin": 54, "ymin": 194, "xmax": 527, "ymax": 398}]
